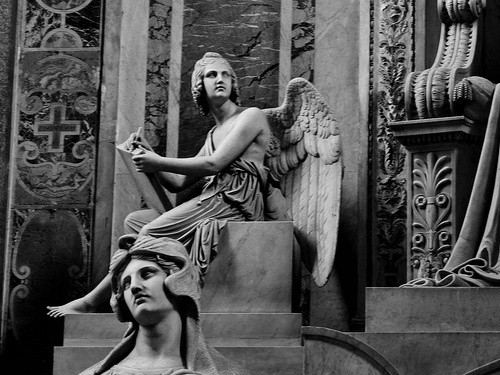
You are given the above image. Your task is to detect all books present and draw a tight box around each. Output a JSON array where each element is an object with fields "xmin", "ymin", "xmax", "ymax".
[{"xmin": 116, "ymin": 133, "xmax": 173, "ymax": 215}]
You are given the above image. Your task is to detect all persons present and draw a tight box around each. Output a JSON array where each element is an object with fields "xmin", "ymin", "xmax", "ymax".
[
  {"xmin": 78, "ymin": 235, "xmax": 250, "ymax": 375},
  {"xmin": 47, "ymin": 51, "xmax": 270, "ymax": 318}
]
